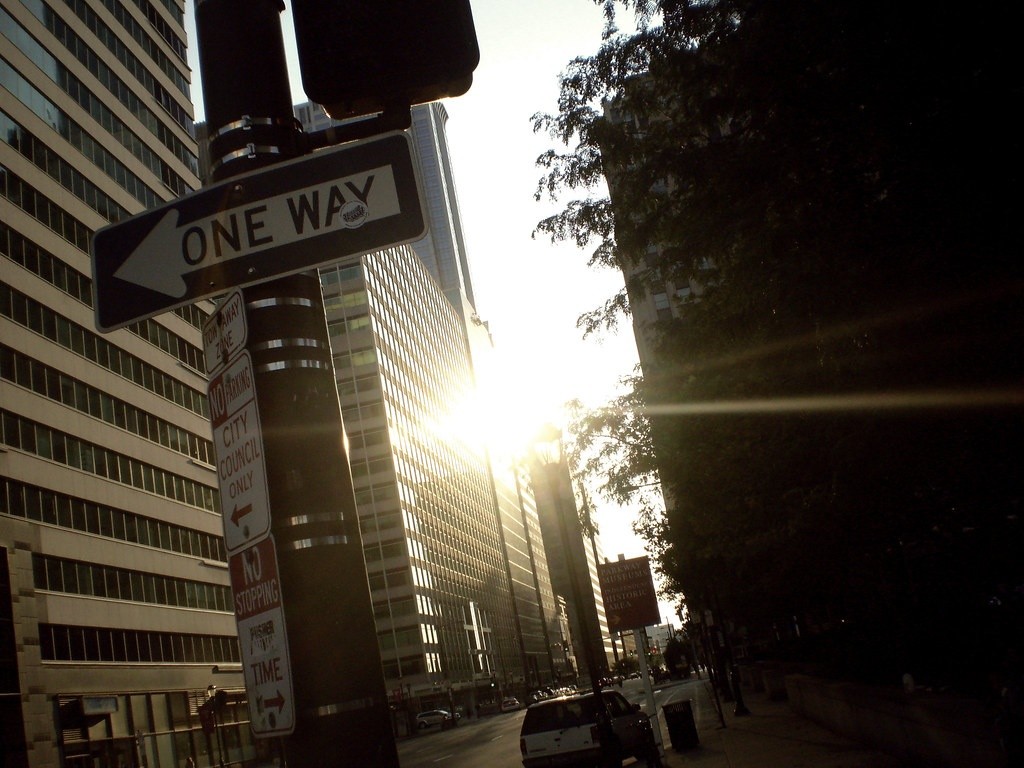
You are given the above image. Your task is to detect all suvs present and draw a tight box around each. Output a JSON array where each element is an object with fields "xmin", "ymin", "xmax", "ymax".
[
  {"xmin": 416, "ymin": 709, "xmax": 461, "ymax": 730},
  {"xmin": 517, "ymin": 686, "xmax": 658, "ymax": 768}
]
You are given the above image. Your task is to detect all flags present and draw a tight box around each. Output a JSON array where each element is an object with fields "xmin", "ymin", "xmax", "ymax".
[{"xmin": 198, "ymin": 704, "xmax": 214, "ymax": 735}]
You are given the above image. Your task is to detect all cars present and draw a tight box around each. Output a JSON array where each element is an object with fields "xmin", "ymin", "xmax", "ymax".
[
  {"xmin": 501, "ymin": 698, "xmax": 521, "ymax": 714},
  {"xmin": 525, "ymin": 684, "xmax": 579, "ymax": 707}
]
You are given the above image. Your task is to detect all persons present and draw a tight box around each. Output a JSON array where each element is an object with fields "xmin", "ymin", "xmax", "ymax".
[{"xmin": 185, "ymin": 757, "xmax": 195, "ymax": 768}]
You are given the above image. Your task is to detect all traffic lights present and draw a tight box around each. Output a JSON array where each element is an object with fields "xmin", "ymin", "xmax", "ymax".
[{"xmin": 563, "ymin": 640, "xmax": 569, "ymax": 652}]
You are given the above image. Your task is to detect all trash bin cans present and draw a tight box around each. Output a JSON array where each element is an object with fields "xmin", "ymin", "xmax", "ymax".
[{"xmin": 661, "ymin": 699, "xmax": 698, "ymax": 752}]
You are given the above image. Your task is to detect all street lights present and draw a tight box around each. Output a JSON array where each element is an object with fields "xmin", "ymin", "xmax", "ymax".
[
  {"xmin": 491, "ymin": 668, "xmax": 499, "ymax": 696},
  {"xmin": 405, "ymin": 682, "xmax": 415, "ymax": 712},
  {"xmin": 508, "ymin": 670, "xmax": 514, "ymax": 693},
  {"xmin": 396, "ymin": 676, "xmax": 410, "ymax": 738},
  {"xmin": 207, "ymin": 683, "xmax": 227, "ymax": 768},
  {"xmin": 529, "ymin": 669, "xmax": 534, "ymax": 685},
  {"xmin": 435, "ymin": 621, "xmax": 464, "ymax": 726}
]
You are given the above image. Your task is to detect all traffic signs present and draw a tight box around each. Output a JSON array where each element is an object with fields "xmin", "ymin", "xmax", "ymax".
[
  {"xmin": 227, "ymin": 534, "xmax": 297, "ymax": 739},
  {"xmin": 87, "ymin": 129, "xmax": 431, "ymax": 336},
  {"xmin": 207, "ymin": 351, "xmax": 274, "ymax": 558}
]
